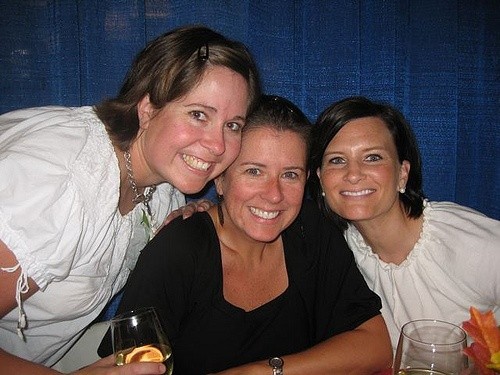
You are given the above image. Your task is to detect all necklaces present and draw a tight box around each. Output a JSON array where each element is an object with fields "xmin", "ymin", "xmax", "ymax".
[{"xmin": 121, "ymin": 142, "xmax": 156, "ymax": 227}]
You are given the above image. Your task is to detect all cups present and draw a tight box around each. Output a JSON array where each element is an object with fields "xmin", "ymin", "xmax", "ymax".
[
  {"xmin": 109, "ymin": 306, "xmax": 174, "ymax": 375},
  {"xmin": 393, "ymin": 319, "xmax": 468, "ymax": 375}
]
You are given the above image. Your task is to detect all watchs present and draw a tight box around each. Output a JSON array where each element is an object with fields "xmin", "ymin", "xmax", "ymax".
[{"xmin": 268, "ymin": 356, "xmax": 284, "ymax": 375}]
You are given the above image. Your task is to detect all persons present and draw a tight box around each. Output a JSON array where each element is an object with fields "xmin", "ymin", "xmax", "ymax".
[
  {"xmin": 99, "ymin": 95, "xmax": 394, "ymax": 375},
  {"xmin": 1, "ymin": 22, "xmax": 259, "ymax": 375},
  {"xmin": 307, "ymin": 95, "xmax": 499, "ymax": 375}
]
